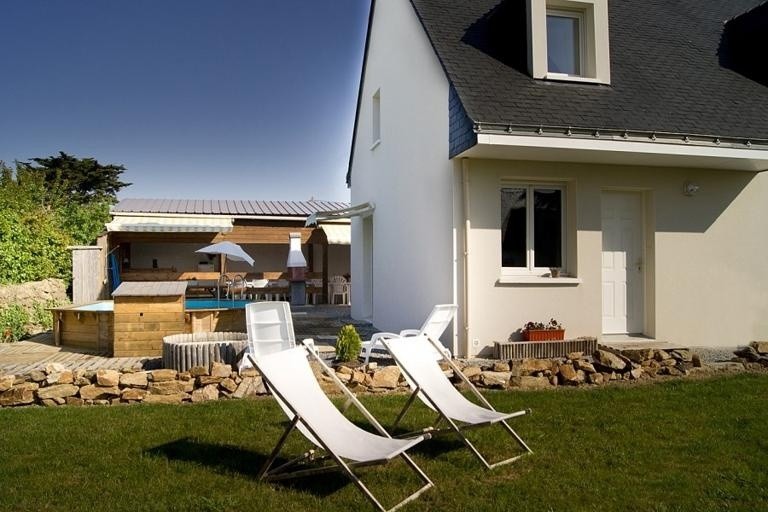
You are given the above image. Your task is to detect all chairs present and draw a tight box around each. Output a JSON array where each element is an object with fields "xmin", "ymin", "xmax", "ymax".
[
  {"xmin": 245, "ymin": 302, "xmax": 319, "ymax": 368},
  {"xmin": 246, "ymin": 339, "xmax": 434, "ymax": 512},
  {"xmin": 226, "ymin": 278, "xmax": 289, "ymax": 301},
  {"xmin": 354, "ymin": 304, "xmax": 459, "ymax": 372},
  {"xmin": 305, "ymin": 275, "xmax": 351, "ymax": 305},
  {"xmin": 378, "ymin": 333, "xmax": 534, "ymax": 471}
]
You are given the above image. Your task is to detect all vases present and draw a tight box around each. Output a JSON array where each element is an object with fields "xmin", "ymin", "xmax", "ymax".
[
  {"xmin": 521, "ymin": 329, "xmax": 565, "ymax": 341},
  {"xmin": 550, "ymin": 269, "xmax": 561, "ymax": 277}
]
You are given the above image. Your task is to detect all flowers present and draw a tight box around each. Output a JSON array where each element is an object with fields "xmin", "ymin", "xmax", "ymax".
[{"xmin": 524, "ymin": 317, "xmax": 562, "ymax": 330}]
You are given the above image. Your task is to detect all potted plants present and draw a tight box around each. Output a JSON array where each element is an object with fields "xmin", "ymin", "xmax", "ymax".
[{"xmin": 331, "ymin": 324, "xmax": 366, "ymax": 375}]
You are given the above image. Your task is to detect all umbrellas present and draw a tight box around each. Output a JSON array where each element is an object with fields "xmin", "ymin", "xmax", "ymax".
[{"xmin": 195, "ymin": 240, "xmax": 256, "ymax": 283}]
